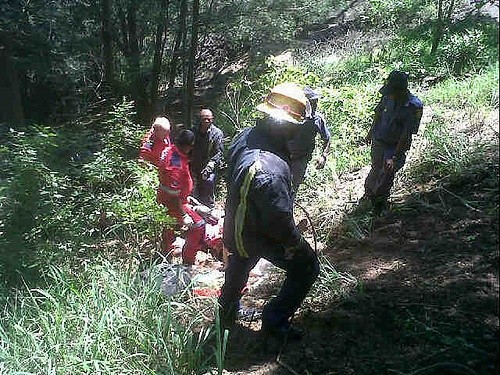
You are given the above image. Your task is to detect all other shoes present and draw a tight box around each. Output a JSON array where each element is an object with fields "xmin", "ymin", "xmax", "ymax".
[
  {"xmin": 358, "ymin": 196, "xmax": 384, "ymax": 215},
  {"xmin": 218, "ymin": 308, "xmax": 257, "ymax": 328},
  {"xmin": 263, "ymin": 327, "xmax": 302, "ymax": 340}
]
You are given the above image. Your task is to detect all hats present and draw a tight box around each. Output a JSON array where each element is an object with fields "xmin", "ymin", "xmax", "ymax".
[
  {"xmin": 257, "ymin": 83, "xmax": 311, "ymax": 125},
  {"xmin": 378, "ymin": 70, "xmax": 408, "ymax": 94}
]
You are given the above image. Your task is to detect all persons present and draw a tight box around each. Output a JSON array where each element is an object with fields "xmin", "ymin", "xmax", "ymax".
[
  {"xmin": 187, "ymin": 108, "xmax": 225, "ymax": 210},
  {"xmin": 156, "ymin": 130, "xmax": 206, "ymax": 269},
  {"xmin": 216, "ymin": 82, "xmax": 319, "ymax": 337},
  {"xmin": 140, "ymin": 117, "xmax": 172, "ymax": 162},
  {"xmin": 364, "ymin": 70, "xmax": 423, "ymax": 211},
  {"xmin": 288, "ymin": 89, "xmax": 331, "ymax": 194}
]
